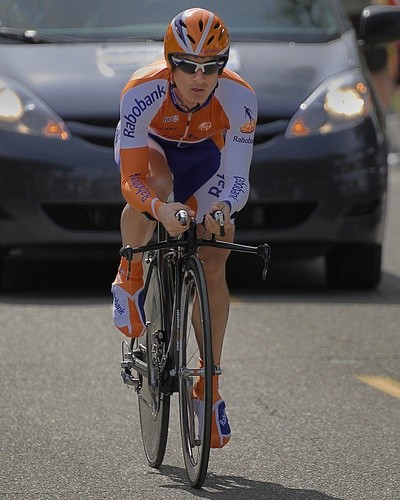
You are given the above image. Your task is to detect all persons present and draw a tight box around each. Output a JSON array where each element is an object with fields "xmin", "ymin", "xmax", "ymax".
[{"xmin": 111, "ymin": 7, "xmax": 257, "ymax": 448}]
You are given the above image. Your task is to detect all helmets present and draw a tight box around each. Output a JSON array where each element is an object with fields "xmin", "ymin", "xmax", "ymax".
[{"xmin": 164, "ymin": 8, "xmax": 230, "ymax": 75}]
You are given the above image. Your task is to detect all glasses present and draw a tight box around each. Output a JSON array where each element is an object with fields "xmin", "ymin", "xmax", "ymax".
[{"xmin": 172, "ymin": 56, "xmax": 225, "ymax": 76}]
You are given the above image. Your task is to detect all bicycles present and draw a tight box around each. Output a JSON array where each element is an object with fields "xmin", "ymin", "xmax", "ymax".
[{"xmin": 120, "ymin": 209, "xmax": 271, "ymax": 489}]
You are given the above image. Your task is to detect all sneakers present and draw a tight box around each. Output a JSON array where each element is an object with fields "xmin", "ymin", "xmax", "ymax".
[
  {"xmin": 192, "ymin": 357, "xmax": 231, "ymax": 448},
  {"xmin": 111, "ymin": 257, "xmax": 147, "ymax": 338}
]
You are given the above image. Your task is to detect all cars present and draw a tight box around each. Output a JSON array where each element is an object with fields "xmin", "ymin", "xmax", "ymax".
[{"xmin": 0, "ymin": 0, "xmax": 400, "ymax": 292}]
